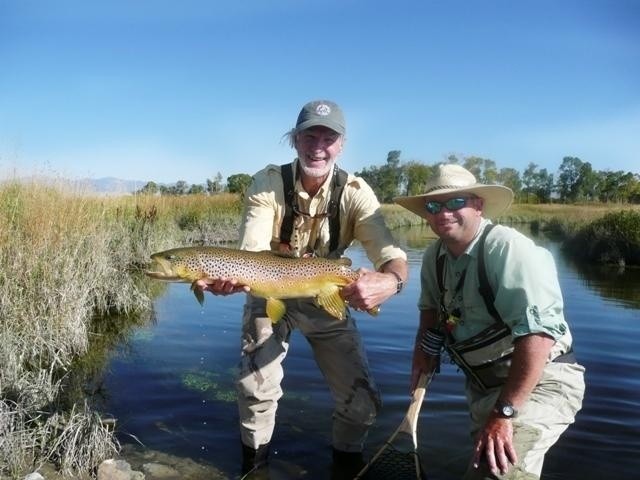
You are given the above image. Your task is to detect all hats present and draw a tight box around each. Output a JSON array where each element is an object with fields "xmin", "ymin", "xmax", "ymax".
[
  {"xmin": 392, "ymin": 164, "xmax": 514, "ymax": 222},
  {"xmin": 294, "ymin": 100, "xmax": 345, "ymax": 137}
]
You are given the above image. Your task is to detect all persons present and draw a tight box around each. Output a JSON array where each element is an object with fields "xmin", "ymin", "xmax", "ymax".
[
  {"xmin": 195, "ymin": 100, "xmax": 409, "ymax": 480},
  {"xmin": 392, "ymin": 162, "xmax": 587, "ymax": 480}
]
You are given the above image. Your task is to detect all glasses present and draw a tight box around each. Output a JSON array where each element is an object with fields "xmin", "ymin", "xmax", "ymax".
[
  {"xmin": 292, "ymin": 192, "xmax": 333, "ymax": 220},
  {"xmin": 425, "ymin": 197, "xmax": 479, "ymax": 215}
]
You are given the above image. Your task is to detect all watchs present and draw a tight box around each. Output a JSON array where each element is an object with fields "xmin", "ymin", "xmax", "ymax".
[
  {"xmin": 495, "ymin": 396, "xmax": 519, "ymax": 420},
  {"xmin": 386, "ymin": 270, "xmax": 404, "ymax": 295}
]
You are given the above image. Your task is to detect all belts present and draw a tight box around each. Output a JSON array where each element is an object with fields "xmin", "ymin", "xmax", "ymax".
[{"xmin": 552, "ymin": 351, "xmax": 577, "ymax": 364}]
